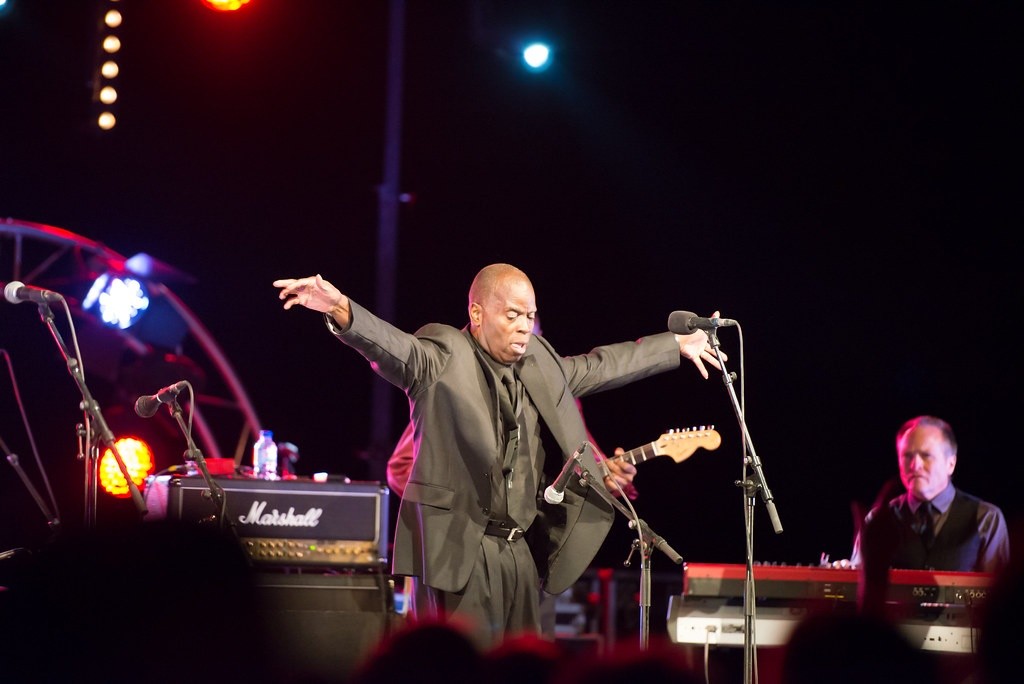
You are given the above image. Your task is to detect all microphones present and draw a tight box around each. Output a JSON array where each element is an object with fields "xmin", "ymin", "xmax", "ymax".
[
  {"xmin": 544, "ymin": 441, "xmax": 588, "ymax": 504},
  {"xmin": 135, "ymin": 379, "xmax": 188, "ymax": 419},
  {"xmin": 4, "ymin": 281, "xmax": 63, "ymax": 304},
  {"xmin": 667, "ymin": 311, "xmax": 738, "ymax": 335}
]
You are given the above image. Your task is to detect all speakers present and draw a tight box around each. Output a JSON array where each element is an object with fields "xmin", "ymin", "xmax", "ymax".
[{"xmin": 256, "ymin": 571, "xmax": 391, "ymax": 679}]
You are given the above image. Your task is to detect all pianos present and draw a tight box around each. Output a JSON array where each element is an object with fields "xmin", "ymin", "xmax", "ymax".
[{"xmin": 682, "ymin": 563, "xmax": 997, "ymax": 609}]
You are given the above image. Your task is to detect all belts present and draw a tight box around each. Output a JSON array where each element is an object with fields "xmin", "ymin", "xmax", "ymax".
[{"xmin": 485, "ymin": 527, "xmax": 524, "ymax": 543}]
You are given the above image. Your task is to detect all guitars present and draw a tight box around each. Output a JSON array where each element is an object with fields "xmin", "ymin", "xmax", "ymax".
[{"xmin": 599, "ymin": 422, "xmax": 722, "ymax": 480}]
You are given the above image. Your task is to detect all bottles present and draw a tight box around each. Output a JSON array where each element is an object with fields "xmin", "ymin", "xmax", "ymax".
[{"xmin": 253, "ymin": 430, "xmax": 278, "ymax": 479}]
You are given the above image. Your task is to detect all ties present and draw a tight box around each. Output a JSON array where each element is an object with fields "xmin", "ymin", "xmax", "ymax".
[
  {"xmin": 920, "ymin": 502, "xmax": 934, "ymax": 550},
  {"xmin": 503, "ymin": 369, "xmax": 537, "ymax": 531}
]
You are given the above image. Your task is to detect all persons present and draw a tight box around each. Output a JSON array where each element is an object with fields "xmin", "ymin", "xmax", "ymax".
[
  {"xmin": 273, "ymin": 263, "xmax": 728, "ymax": 653},
  {"xmin": 851, "ymin": 416, "xmax": 1010, "ymax": 626},
  {"xmin": 0, "ymin": 519, "xmax": 1024, "ymax": 684}
]
